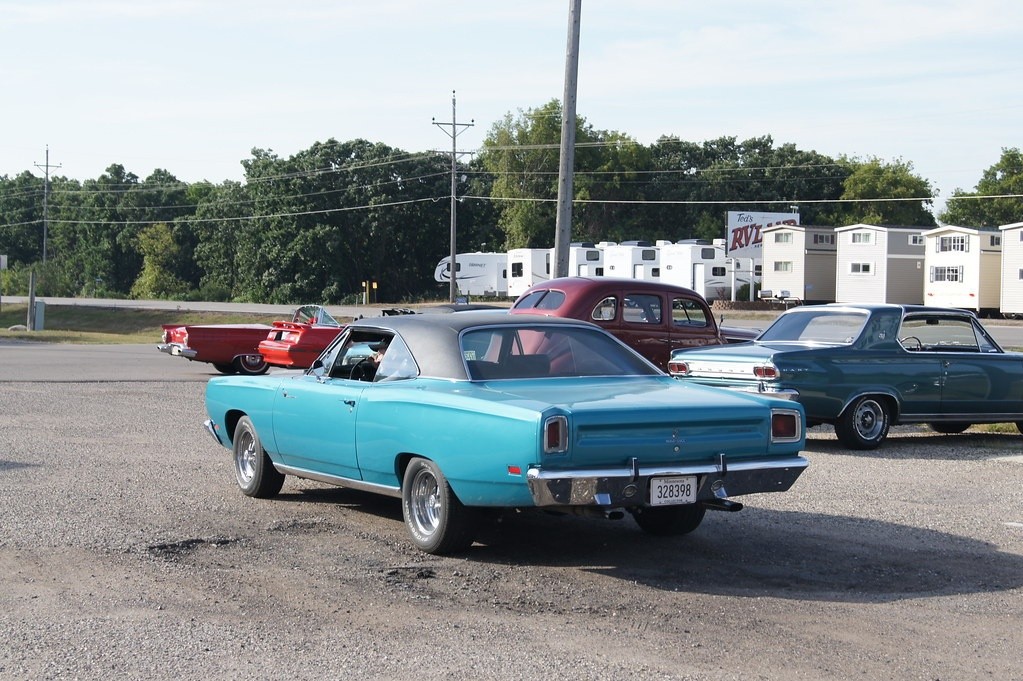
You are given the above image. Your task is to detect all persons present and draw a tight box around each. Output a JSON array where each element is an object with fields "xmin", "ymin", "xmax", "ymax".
[{"xmin": 365, "ymin": 338, "xmax": 392, "ymax": 369}]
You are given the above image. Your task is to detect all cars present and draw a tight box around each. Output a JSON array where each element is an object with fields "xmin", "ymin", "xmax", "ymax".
[
  {"xmin": 201, "ymin": 311, "xmax": 809, "ymax": 556},
  {"xmin": 342, "ymin": 305, "xmax": 509, "ymax": 366},
  {"xmin": 480, "ymin": 274, "xmax": 764, "ymax": 377},
  {"xmin": 668, "ymin": 300, "xmax": 1023, "ymax": 451}
]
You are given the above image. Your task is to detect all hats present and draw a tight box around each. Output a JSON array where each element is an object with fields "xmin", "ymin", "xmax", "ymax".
[{"xmin": 368, "ymin": 337, "xmax": 400, "ymax": 352}]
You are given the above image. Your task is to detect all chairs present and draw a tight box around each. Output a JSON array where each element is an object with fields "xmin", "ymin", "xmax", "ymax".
[
  {"xmin": 497, "ymin": 354, "xmax": 551, "ymax": 375},
  {"xmin": 467, "ymin": 360, "xmax": 499, "ymax": 379}
]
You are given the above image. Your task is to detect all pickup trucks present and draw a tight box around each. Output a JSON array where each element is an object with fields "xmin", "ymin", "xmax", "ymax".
[{"xmin": 156, "ymin": 305, "xmax": 418, "ymax": 376}]
